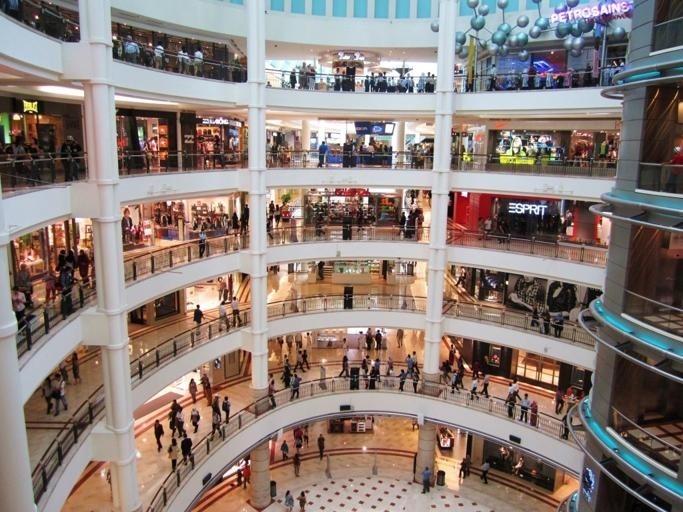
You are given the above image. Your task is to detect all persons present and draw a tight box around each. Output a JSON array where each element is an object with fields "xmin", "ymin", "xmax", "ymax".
[
  {"xmin": 270, "ymin": 260, "xmax": 416, "ymax": 312},
  {"xmin": 450, "ymin": 263, "xmax": 564, "ymax": 338},
  {"xmin": 465, "ymin": 59, "xmax": 622, "ymax": 92},
  {"xmin": 284, "ymin": 489, "xmax": 294, "ymax": 512},
  {"xmin": 420, "ymin": 466, "xmax": 432, "ymax": 494},
  {"xmin": 193, "ymin": 274, "xmax": 242, "ymax": 335},
  {"xmin": 268, "ymin": 330, "xmax": 420, "ymax": 400},
  {"xmin": 113, "ymin": 33, "xmax": 245, "ymax": 83},
  {"xmin": 265, "ymin": 190, "xmax": 432, "ymax": 241},
  {"xmin": 456, "ymin": 140, "xmax": 616, "ymax": 171},
  {"xmin": 2, "ymin": 2, "xmax": 80, "ymax": 40},
  {"xmin": 480, "ymin": 457, "xmax": 489, "ymax": 484},
  {"xmin": 119, "ymin": 134, "xmax": 239, "ymax": 170},
  {"xmin": 475, "ymin": 206, "xmax": 573, "ymax": 244},
  {"xmin": 264, "ymin": 136, "xmax": 433, "ymax": 169},
  {"xmin": 1, "ymin": 133, "xmax": 81, "ymax": 187},
  {"xmin": 41, "ymin": 350, "xmax": 82, "ymax": 416},
  {"xmin": 267, "ymin": 62, "xmax": 435, "ymax": 93},
  {"xmin": 122, "ymin": 202, "xmax": 249, "ymax": 257},
  {"xmin": 153, "ymin": 372, "xmax": 230, "ymax": 472},
  {"xmin": 440, "ymin": 345, "xmax": 586, "ymax": 440},
  {"xmin": 12, "ymin": 246, "xmax": 91, "ymax": 334},
  {"xmin": 457, "ymin": 457, "xmax": 468, "ymax": 478},
  {"xmin": 241, "ymin": 463, "xmax": 250, "ymax": 489},
  {"xmin": 235, "ymin": 466, "xmax": 241, "ymax": 486},
  {"xmin": 246, "ymin": 416, "xmax": 543, "ymax": 476},
  {"xmin": 295, "ymin": 490, "xmax": 308, "ymax": 511}
]
